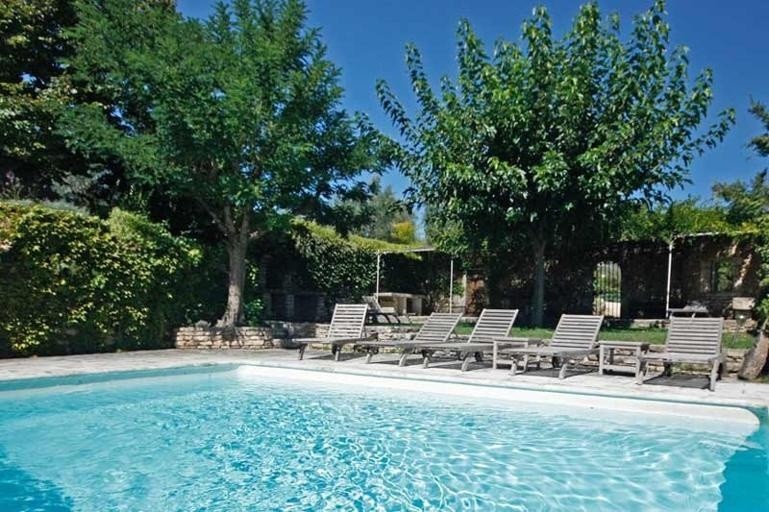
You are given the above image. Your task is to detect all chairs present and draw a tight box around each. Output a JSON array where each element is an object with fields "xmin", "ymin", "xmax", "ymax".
[{"xmin": 290, "ymin": 295, "xmax": 727, "ymax": 391}]
